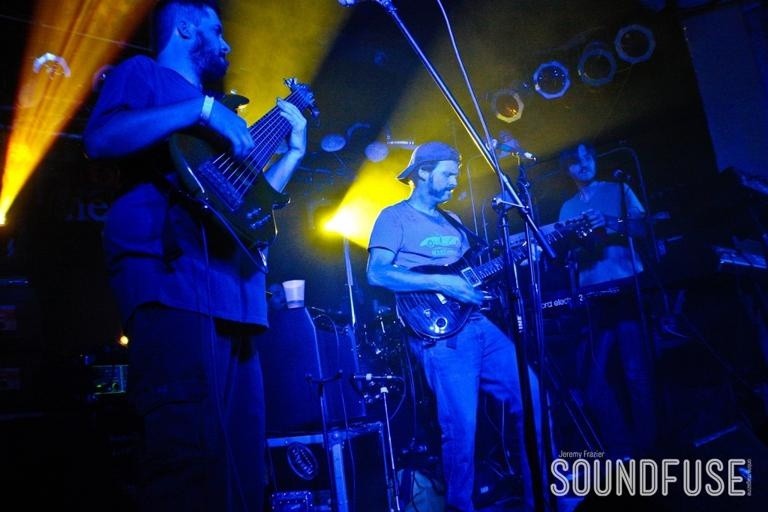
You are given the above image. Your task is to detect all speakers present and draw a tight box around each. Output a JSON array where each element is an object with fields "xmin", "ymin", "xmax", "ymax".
[{"xmin": 258, "ymin": 305, "xmax": 374, "ymax": 436}]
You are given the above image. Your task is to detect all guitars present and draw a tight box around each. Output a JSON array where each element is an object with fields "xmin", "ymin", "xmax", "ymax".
[
  {"xmin": 168, "ymin": 74, "xmax": 320, "ymax": 248},
  {"xmin": 394, "ymin": 213, "xmax": 592, "ymax": 343}
]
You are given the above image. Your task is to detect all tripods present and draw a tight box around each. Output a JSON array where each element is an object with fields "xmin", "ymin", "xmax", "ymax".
[{"xmin": 374, "ymin": 1, "xmax": 608, "ymax": 512}]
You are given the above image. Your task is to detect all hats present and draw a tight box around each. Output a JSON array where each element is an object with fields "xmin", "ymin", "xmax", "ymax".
[{"xmin": 397, "ymin": 142, "xmax": 461, "ymax": 184}]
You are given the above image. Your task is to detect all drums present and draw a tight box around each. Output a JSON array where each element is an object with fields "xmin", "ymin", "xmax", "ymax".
[{"xmin": 363, "ymin": 305, "xmax": 407, "ymax": 361}]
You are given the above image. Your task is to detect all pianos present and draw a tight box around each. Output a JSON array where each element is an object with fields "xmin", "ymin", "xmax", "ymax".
[{"xmin": 524, "ymin": 272, "xmax": 654, "ymax": 319}]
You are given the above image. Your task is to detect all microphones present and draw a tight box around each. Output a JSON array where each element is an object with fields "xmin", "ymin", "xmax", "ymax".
[
  {"xmin": 485, "ymin": 139, "xmax": 533, "ymax": 159},
  {"xmin": 612, "ymin": 169, "xmax": 633, "ymax": 182},
  {"xmin": 338, "ymin": 0, "xmax": 360, "ymax": 8},
  {"xmin": 352, "ymin": 373, "xmax": 398, "ymax": 381}
]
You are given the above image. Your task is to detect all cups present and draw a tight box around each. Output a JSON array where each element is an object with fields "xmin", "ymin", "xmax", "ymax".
[{"xmin": 282, "ymin": 279, "xmax": 306, "ymax": 308}]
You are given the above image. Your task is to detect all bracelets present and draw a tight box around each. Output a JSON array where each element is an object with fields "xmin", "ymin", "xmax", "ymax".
[{"xmin": 198, "ymin": 95, "xmax": 215, "ymax": 129}]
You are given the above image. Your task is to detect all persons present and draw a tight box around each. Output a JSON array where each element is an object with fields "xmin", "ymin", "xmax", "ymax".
[
  {"xmin": 363, "ymin": 138, "xmax": 563, "ymax": 511},
  {"xmin": 78, "ymin": 1, "xmax": 310, "ymax": 511},
  {"xmin": 554, "ymin": 143, "xmax": 663, "ymax": 469}
]
